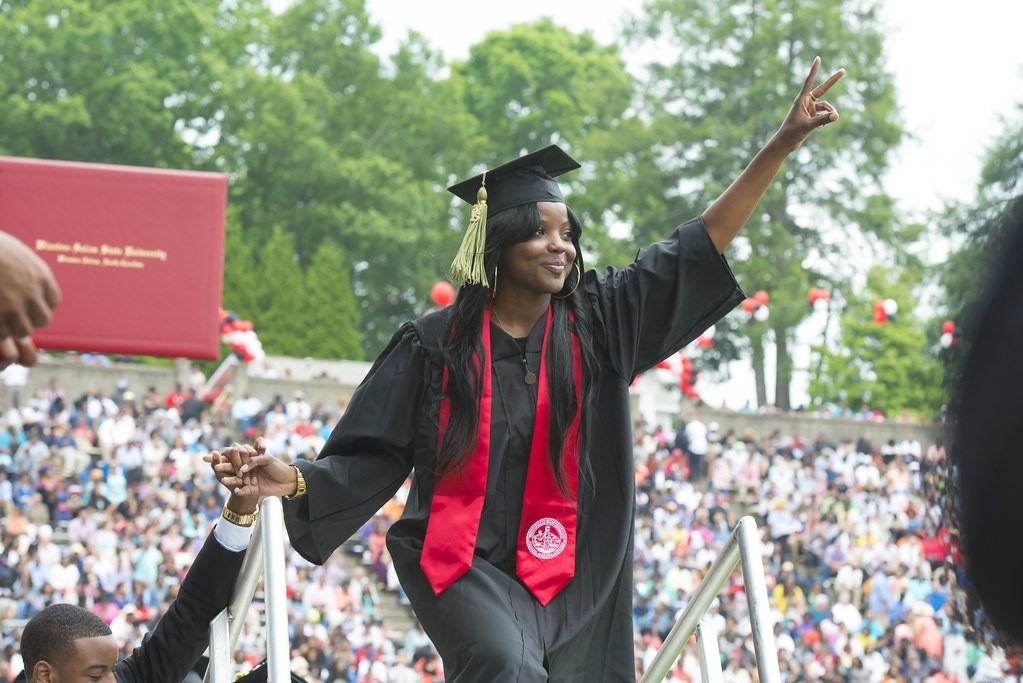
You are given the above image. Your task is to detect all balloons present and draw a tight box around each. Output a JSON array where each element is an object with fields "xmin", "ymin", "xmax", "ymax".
[
  {"xmin": 940, "ymin": 320, "xmax": 962, "ymax": 349},
  {"xmin": 657, "ymin": 350, "xmax": 701, "ymax": 398},
  {"xmin": 808, "ymin": 288, "xmax": 829, "ymax": 311},
  {"xmin": 431, "ymin": 281, "xmax": 454, "ymax": 305},
  {"xmin": 220, "ymin": 308, "xmax": 266, "ymax": 363},
  {"xmin": 697, "ymin": 325, "xmax": 716, "ymax": 347},
  {"xmin": 743, "ymin": 290, "xmax": 770, "ymax": 321},
  {"xmin": 874, "ymin": 298, "xmax": 897, "ymax": 324}
]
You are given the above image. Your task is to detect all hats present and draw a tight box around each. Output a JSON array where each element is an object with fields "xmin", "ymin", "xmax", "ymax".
[{"xmin": 446, "ymin": 143, "xmax": 582, "ymax": 290}]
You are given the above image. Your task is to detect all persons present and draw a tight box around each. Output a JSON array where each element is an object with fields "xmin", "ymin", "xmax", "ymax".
[
  {"xmin": 0, "ymin": 378, "xmax": 1023, "ymax": 683},
  {"xmin": 0, "ymin": 230, "xmax": 61, "ymax": 374},
  {"xmin": 12, "ymin": 435, "xmax": 266, "ymax": 683},
  {"xmin": 204, "ymin": 56, "xmax": 845, "ymax": 683}
]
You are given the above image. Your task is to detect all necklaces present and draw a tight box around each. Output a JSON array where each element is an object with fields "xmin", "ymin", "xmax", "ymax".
[{"xmin": 493, "ymin": 307, "xmax": 504, "ymax": 331}]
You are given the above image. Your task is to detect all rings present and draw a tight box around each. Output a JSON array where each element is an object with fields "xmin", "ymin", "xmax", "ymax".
[{"xmin": 810, "ymin": 92, "xmax": 818, "ymax": 100}]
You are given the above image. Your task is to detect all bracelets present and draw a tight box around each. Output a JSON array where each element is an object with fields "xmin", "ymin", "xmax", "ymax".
[
  {"xmin": 285, "ymin": 466, "xmax": 306, "ymax": 501},
  {"xmin": 223, "ymin": 501, "xmax": 259, "ymax": 525}
]
